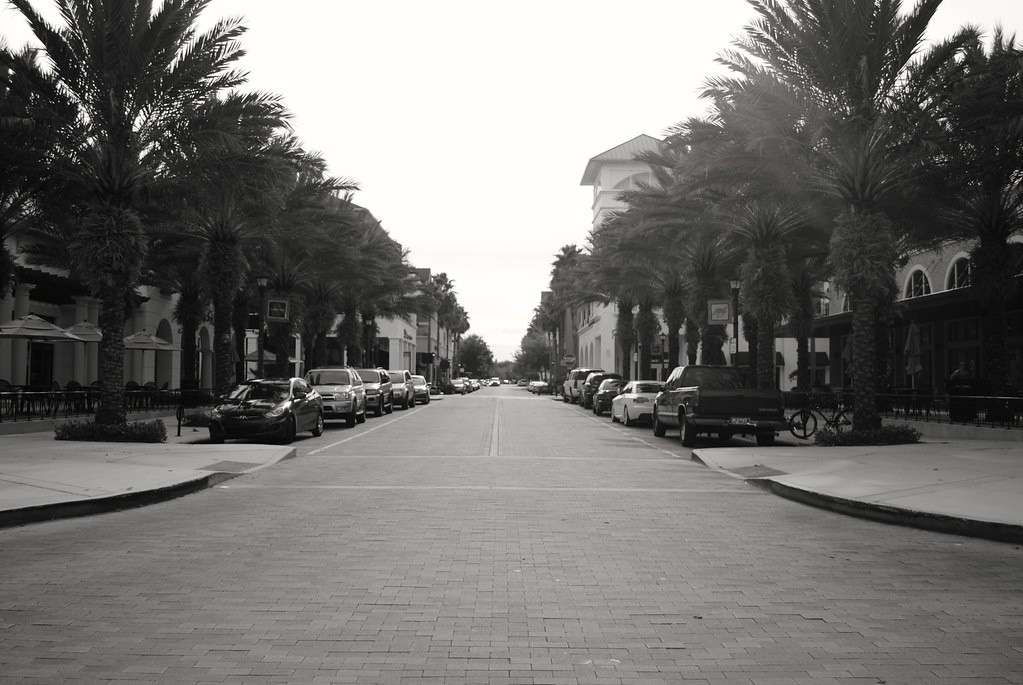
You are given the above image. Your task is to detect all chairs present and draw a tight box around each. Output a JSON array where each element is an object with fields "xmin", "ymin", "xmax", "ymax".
[{"xmin": 0, "ymin": 379, "xmax": 169, "ymax": 417}]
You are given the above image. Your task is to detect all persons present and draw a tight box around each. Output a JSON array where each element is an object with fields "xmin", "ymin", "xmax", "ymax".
[{"xmin": 949, "ymin": 361, "xmax": 969, "ymax": 380}]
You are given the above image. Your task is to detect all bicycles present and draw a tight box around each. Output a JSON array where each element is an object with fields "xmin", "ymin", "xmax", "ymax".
[{"xmin": 790, "ymin": 397, "xmax": 855, "ymax": 439}]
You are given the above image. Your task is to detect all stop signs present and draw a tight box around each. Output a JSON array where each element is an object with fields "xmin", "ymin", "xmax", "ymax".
[{"xmin": 563, "ymin": 353, "xmax": 577, "ymax": 366}]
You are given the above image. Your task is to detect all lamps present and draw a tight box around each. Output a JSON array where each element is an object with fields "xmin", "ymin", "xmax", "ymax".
[{"xmin": 177, "ymin": 406, "xmax": 184, "ymax": 419}]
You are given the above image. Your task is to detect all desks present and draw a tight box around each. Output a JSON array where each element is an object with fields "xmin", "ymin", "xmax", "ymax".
[
  {"xmin": 12, "ymin": 385, "xmax": 48, "ymax": 416},
  {"xmin": 63, "ymin": 386, "xmax": 98, "ymax": 412},
  {"xmin": 131, "ymin": 385, "xmax": 154, "ymax": 411}
]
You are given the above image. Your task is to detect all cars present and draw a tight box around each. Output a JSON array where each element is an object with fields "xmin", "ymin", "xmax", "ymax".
[
  {"xmin": 593, "ymin": 379, "xmax": 629, "ymax": 415},
  {"xmin": 450, "ymin": 379, "xmax": 467, "ymax": 395},
  {"xmin": 411, "ymin": 375, "xmax": 432, "ymax": 404},
  {"xmin": 386, "ymin": 370, "xmax": 416, "ymax": 410},
  {"xmin": 208, "ymin": 377, "xmax": 324, "ymax": 444},
  {"xmin": 581, "ymin": 372, "xmax": 622, "ymax": 409},
  {"xmin": 611, "ymin": 380, "xmax": 666, "ymax": 428},
  {"xmin": 511, "ymin": 379, "xmax": 548, "ymax": 392},
  {"xmin": 457, "ymin": 377, "xmax": 490, "ymax": 392},
  {"xmin": 503, "ymin": 380, "xmax": 509, "ymax": 384},
  {"xmin": 489, "ymin": 377, "xmax": 500, "ymax": 387}
]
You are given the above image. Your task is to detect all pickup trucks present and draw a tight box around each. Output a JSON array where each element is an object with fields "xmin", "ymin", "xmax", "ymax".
[{"xmin": 654, "ymin": 365, "xmax": 793, "ymax": 448}]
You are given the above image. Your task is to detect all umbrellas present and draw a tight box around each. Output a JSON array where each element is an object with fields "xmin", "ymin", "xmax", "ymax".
[
  {"xmin": 904, "ymin": 321, "xmax": 920, "ymax": 388},
  {"xmin": 0, "ymin": 314, "xmax": 81, "ymax": 386},
  {"xmin": 244, "ymin": 349, "xmax": 303, "ymax": 366},
  {"xmin": 841, "ymin": 334, "xmax": 856, "ymax": 389},
  {"xmin": 122, "ymin": 330, "xmax": 184, "ymax": 386},
  {"xmin": 43, "ymin": 321, "xmax": 105, "ymax": 384}
]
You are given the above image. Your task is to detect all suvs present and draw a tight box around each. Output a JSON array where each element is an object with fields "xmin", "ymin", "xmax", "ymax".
[
  {"xmin": 304, "ymin": 366, "xmax": 365, "ymax": 427},
  {"xmin": 354, "ymin": 367, "xmax": 393, "ymax": 417},
  {"xmin": 562, "ymin": 368, "xmax": 605, "ymax": 404}
]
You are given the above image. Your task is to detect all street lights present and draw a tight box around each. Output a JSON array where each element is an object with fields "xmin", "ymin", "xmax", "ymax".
[
  {"xmin": 255, "ymin": 270, "xmax": 270, "ymax": 380},
  {"xmin": 364, "ymin": 313, "xmax": 376, "ymax": 369},
  {"xmin": 729, "ymin": 273, "xmax": 741, "ymax": 365},
  {"xmin": 660, "ymin": 332, "xmax": 666, "ymax": 379}
]
